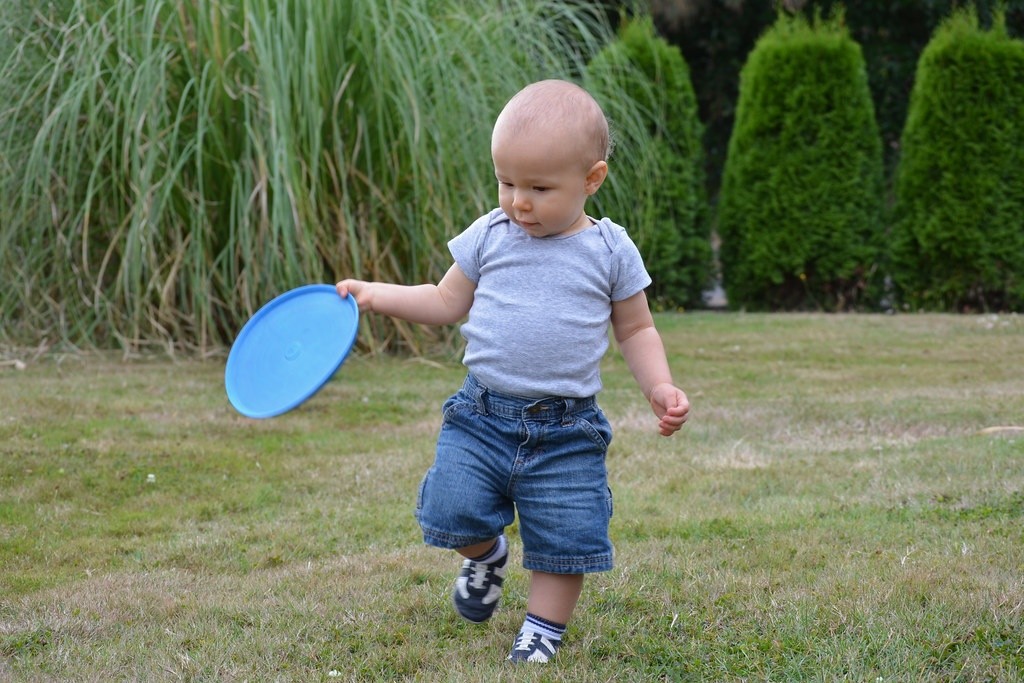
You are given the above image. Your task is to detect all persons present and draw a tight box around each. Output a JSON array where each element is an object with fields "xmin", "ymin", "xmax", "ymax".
[{"xmin": 334, "ymin": 79, "xmax": 691, "ymax": 664}]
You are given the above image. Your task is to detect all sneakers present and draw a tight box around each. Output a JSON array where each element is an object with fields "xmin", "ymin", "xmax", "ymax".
[
  {"xmin": 453, "ymin": 537, "xmax": 509, "ymax": 623},
  {"xmin": 506, "ymin": 628, "xmax": 560, "ymax": 664}
]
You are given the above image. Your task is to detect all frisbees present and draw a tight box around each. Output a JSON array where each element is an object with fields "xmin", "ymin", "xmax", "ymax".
[{"xmin": 224, "ymin": 282, "xmax": 361, "ymax": 420}]
